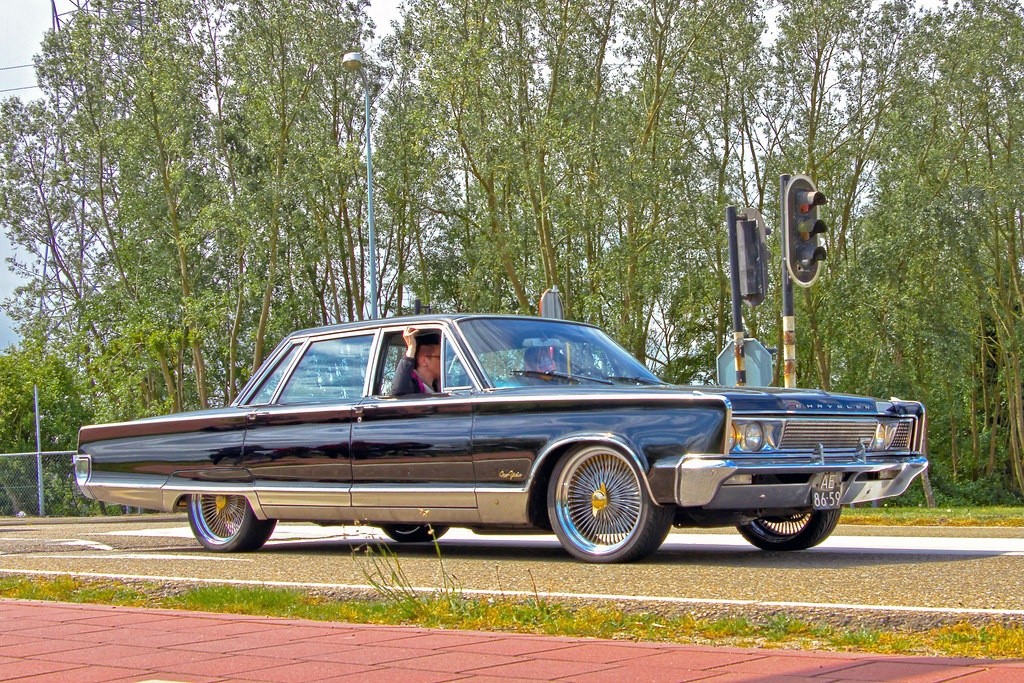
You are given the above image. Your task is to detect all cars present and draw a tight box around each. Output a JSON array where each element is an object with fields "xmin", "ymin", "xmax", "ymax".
[{"xmin": 70, "ymin": 312, "xmax": 929, "ymax": 564}]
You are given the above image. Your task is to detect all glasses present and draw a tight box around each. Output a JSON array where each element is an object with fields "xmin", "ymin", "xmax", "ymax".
[{"xmin": 423, "ymin": 354, "xmax": 442, "ymax": 359}]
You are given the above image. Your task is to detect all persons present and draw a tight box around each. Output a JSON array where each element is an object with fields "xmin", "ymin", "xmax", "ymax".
[
  {"xmin": 391, "ymin": 326, "xmax": 441, "ymax": 396},
  {"xmin": 508, "ymin": 347, "xmax": 559, "ymax": 387}
]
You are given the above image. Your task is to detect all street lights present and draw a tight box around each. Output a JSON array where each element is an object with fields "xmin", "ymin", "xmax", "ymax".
[{"xmin": 341, "ymin": 49, "xmax": 381, "ymax": 321}]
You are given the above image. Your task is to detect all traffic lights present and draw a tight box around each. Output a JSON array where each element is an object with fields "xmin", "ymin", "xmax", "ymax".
[{"xmin": 782, "ymin": 173, "xmax": 829, "ymax": 288}]
